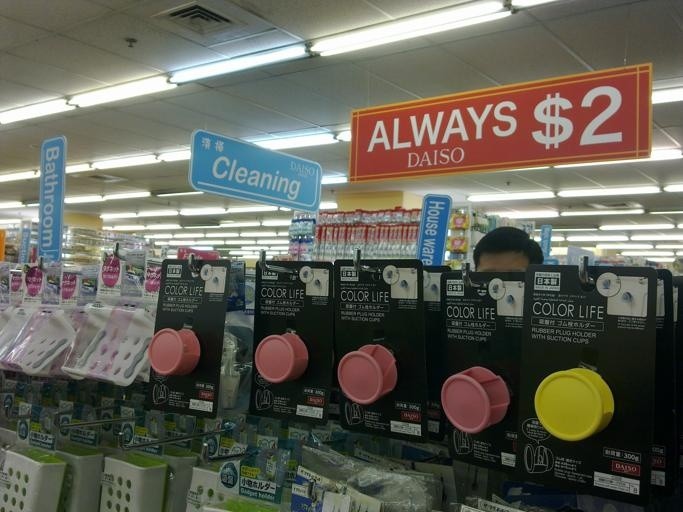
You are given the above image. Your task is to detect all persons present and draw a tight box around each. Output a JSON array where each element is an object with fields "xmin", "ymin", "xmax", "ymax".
[{"xmin": 472, "ymin": 226, "xmax": 543, "ymax": 273}]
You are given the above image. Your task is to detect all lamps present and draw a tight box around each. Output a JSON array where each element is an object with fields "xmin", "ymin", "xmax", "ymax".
[
  {"xmin": 310, "ymin": 0, "xmax": 555, "ymax": 57},
  {"xmin": 241, "ymin": 126, "xmax": 352, "ymax": 148},
  {"xmin": 652, "ymin": 86, "xmax": 683, "ymax": 106},
  {"xmin": 1, "ymin": 148, "xmax": 683, "ymax": 264},
  {"xmin": 1, "ymin": 95, "xmax": 79, "ymax": 125},
  {"xmin": 169, "ymin": 41, "xmax": 310, "ymax": 86},
  {"xmin": 68, "ymin": 72, "xmax": 179, "ymax": 109}
]
